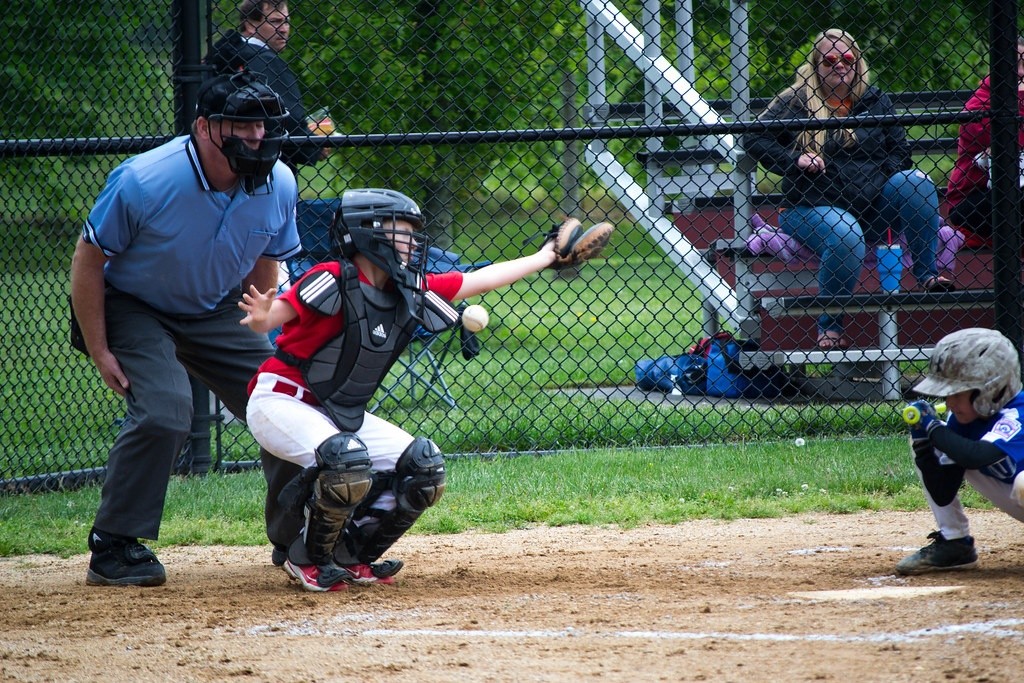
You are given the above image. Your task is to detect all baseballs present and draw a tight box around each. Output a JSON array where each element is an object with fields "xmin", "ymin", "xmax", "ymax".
[{"xmin": 461, "ymin": 304, "xmax": 490, "ymax": 332}]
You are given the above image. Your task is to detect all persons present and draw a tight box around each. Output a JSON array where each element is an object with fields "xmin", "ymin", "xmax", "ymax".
[
  {"xmin": 895, "ymin": 328, "xmax": 1024, "ymax": 572},
  {"xmin": 947, "ymin": 36, "xmax": 1024, "ymax": 284},
  {"xmin": 71, "ymin": 72, "xmax": 302, "ymax": 585},
  {"xmin": 238, "ymin": 188, "xmax": 615, "ymax": 592},
  {"xmin": 200, "ymin": 0, "xmax": 332, "ymax": 201},
  {"xmin": 741, "ymin": 29, "xmax": 953, "ymax": 348}
]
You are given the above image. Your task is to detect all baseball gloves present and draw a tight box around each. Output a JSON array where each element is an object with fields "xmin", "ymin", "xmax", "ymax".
[{"xmin": 518, "ymin": 216, "xmax": 615, "ymax": 271}]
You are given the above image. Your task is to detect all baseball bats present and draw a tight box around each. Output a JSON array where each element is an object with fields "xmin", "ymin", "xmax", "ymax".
[{"xmin": 902, "ymin": 400, "xmax": 946, "ymax": 425}]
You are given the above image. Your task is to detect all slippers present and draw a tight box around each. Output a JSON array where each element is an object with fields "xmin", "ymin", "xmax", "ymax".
[
  {"xmin": 816, "ymin": 329, "xmax": 849, "ymax": 351},
  {"xmin": 920, "ymin": 273, "xmax": 957, "ymax": 292}
]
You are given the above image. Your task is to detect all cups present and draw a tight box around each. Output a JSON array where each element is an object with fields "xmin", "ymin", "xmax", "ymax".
[{"xmin": 878, "ymin": 245, "xmax": 902, "ymax": 293}]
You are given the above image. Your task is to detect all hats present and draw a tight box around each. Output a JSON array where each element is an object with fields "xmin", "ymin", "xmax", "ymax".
[{"xmin": 195, "ymin": 77, "xmax": 274, "ymax": 122}]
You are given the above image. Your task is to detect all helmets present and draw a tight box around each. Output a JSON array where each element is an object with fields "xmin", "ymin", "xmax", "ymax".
[
  {"xmin": 329, "ymin": 188, "xmax": 426, "ymax": 260},
  {"xmin": 914, "ymin": 327, "xmax": 1023, "ymax": 417}
]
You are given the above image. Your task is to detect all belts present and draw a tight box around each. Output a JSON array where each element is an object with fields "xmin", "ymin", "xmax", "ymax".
[{"xmin": 272, "ymin": 381, "xmax": 325, "ymax": 408}]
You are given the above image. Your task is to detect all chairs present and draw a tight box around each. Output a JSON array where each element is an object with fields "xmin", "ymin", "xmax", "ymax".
[{"xmin": 288, "ymin": 199, "xmax": 493, "ymax": 411}]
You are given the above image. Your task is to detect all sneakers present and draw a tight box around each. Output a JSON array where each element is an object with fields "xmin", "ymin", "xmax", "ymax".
[
  {"xmin": 282, "ymin": 554, "xmax": 348, "ymax": 591},
  {"xmin": 272, "ymin": 543, "xmax": 290, "ymax": 564},
  {"xmin": 85, "ymin": 527, "xmax": 167, "ymax": 586},
  {"xmin": 895, "ymin": 531, "xmax": 978, "ymax": 573},
  {"xmin": 328, "ymin": 557, "xmax": 395, "ymax": 584}
]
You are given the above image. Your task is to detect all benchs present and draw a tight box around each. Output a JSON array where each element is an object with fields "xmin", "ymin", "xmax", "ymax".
[{"xmin": 584, "ymin": 83, "xmax": 995, "ymax": 402}]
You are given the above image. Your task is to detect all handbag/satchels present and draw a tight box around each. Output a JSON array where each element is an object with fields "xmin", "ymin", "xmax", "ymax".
[{"xmin": 634, "ymin": 330, "xmax": 787, "ymax": 399}]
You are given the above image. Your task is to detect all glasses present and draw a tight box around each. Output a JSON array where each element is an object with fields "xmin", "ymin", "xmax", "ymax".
[{"xmin": 819, "ymin": 54, "xmax": 857, "ymax": 67}]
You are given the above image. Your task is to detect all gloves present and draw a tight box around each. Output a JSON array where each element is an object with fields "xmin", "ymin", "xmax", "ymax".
[{"xmin": 903, "ymin": 399, "xmax": 943, "ymax": 443}]
